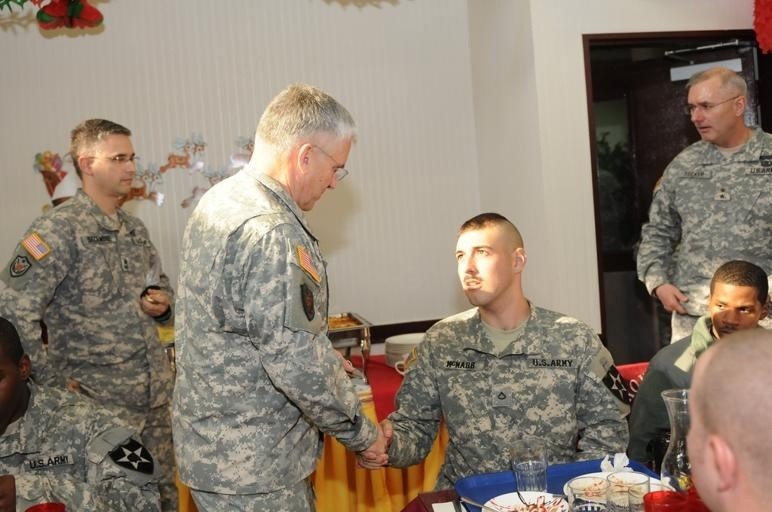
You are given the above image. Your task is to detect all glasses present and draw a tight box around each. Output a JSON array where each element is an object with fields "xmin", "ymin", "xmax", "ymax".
[
  {"xmin": 299, "ymin": 141, "xmax": 348, "ymax": 182},
  {"xmin": 86, "ymin": 155, "xmax": 140, "ymax": 164},
  {"xmin": 684, "ymin": 94, "xmax": 740, "ymax": 116}
]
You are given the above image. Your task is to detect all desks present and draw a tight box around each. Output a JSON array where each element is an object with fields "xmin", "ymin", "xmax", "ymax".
[
  {"xmin": 398, "ymin": 457, "xmax": 688, "ymax": 512},
  {"xmin": 176, "ymin": 356, "xmax": 455, "ymax": 512}
]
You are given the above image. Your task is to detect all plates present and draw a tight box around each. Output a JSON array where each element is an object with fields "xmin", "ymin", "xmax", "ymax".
[
  {"xmin": 481, "ymin": 491, "xmax": 568, "ymax": 512},
  {"xmin": 384, "ymin": 333, "xmax": 428, "ymax": 366},
  {"xmin": 563, "ymin": 471, "xmax": 677, "ymax": 507}
]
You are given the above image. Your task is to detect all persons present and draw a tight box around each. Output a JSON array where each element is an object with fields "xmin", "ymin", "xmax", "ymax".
[
  {"xmin": 623, "ymin": 261, "xmax": 772, "ymax": 478},
  {"xmin": 168, "ymin": 85, "xmax": 387, "ymax": 511},
  {"xmin": 352, "ymin": 211, "xmax": 630, "ymax": 490},
  {"xmin": 688, "ymin": 326, "xmax": 772, "ymax": 511},
  {"xmin": 631, "ymin": 67, "xmax": 771, "ymax": 342},
  {"xmin": 0, "ymin": 315, "xmax": 164, "ymax": 512},
  {"xmin": 0, "ymin": 118, "xmax": 179, "ymax": 512}
]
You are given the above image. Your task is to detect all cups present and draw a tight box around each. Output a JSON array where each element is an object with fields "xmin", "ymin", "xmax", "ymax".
[
  {"xmin": 510, "ymin": 437, "xmax": 548, "ymax": 491},
  {"xmin": 393, "ymin": 354, "xmax": 408, "ymax": 379},
  {"xmin": 659, "ymin": 389, "xmax": 696, "ymax": 489},
  {"xmin": 568, "ymin": 470, "xmax": 710, "ymax": 512}
]
